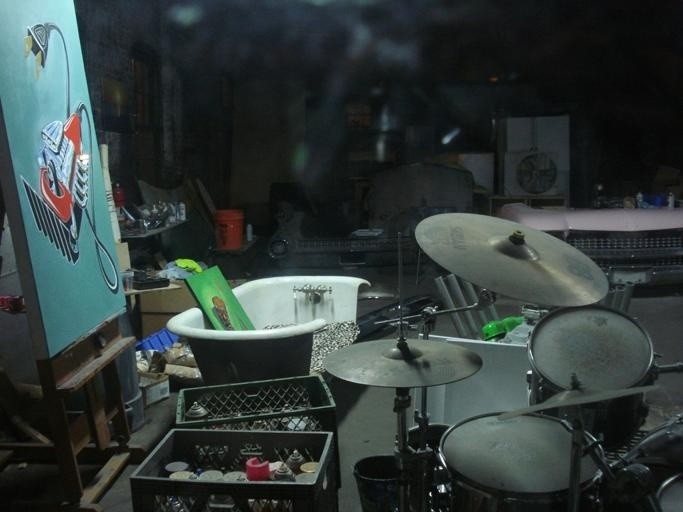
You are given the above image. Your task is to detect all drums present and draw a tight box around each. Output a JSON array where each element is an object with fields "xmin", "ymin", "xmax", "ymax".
[
  {"xmin": 575, "ymin": 412, "xmax": 682, "ymax": 512},
  {"xmin": 432, "ymin": 411, "xmax": 604, "ymax": 508},
  {"xmin": 527, "ymin": 305, "xmax": 656, "ymax": 450}
]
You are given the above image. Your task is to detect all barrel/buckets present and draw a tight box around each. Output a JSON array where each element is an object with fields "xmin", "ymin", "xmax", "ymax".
[{"xmin": 213, "ymin": 209, "xmax": 246, "ymax": 248}]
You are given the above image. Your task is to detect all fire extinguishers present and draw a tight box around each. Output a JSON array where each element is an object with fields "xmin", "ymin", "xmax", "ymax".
[{"xmin": 112, "ymin": 182, "xmax": 127, "ymax": 225}]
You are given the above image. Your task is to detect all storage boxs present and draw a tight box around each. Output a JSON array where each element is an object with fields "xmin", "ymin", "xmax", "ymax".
[{"xmin": 139, "ymin": 278, "xmax": 199, "ymax": 342}]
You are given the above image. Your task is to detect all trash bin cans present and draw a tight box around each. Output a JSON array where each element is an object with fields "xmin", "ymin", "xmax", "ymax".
[{"xmin": 354, "ymin": 455, "xmax": 400, "ymax": 512}]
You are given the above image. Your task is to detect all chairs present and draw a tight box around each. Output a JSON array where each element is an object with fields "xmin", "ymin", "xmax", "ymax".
[{"xmin": 347, "ymin": 163, "xmax": 474, "ymax": 266}]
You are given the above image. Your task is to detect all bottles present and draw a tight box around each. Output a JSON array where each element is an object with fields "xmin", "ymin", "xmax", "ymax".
[
  {"xmin": 246, "ymin": 224, "xmax": 253, "ymax": 241},
  {"xmin": 667, "ymin": 193, "xmax": 675, "ymax": 208},
  {"xmin": 275, "ymin": 449, "xmax": 306, "ymax": 481},
  {"xmin": 114, "ymin": 183, "xmax": 125, "ymax": 208},
  {"xmin": 636, "ymin": 192, "xmax": 643, "ymax": 208}
]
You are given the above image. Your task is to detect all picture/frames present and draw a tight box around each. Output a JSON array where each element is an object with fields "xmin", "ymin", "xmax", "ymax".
[{"xmin": 98, "ymin": 75, "xmax": 131, "ymax": 130}]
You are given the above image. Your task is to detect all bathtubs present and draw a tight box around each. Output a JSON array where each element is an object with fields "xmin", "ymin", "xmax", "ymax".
[{"xmin": 165, "ymin": 276, "xmax": 373, "ymax": 386}]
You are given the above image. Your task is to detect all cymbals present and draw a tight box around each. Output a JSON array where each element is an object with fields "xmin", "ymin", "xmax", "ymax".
[
  {"xmin": 323, "ymin": 338, "xmax": 481, "ymax": 387},
  {"xmin": 415, "ymin": 213, "xmax": 609, "ymax": 307}
]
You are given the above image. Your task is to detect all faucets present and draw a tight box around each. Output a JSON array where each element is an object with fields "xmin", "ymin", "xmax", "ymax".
[{"xmin": 293, "ymin": 280, "xmax": 332, "ymax": 303}]
